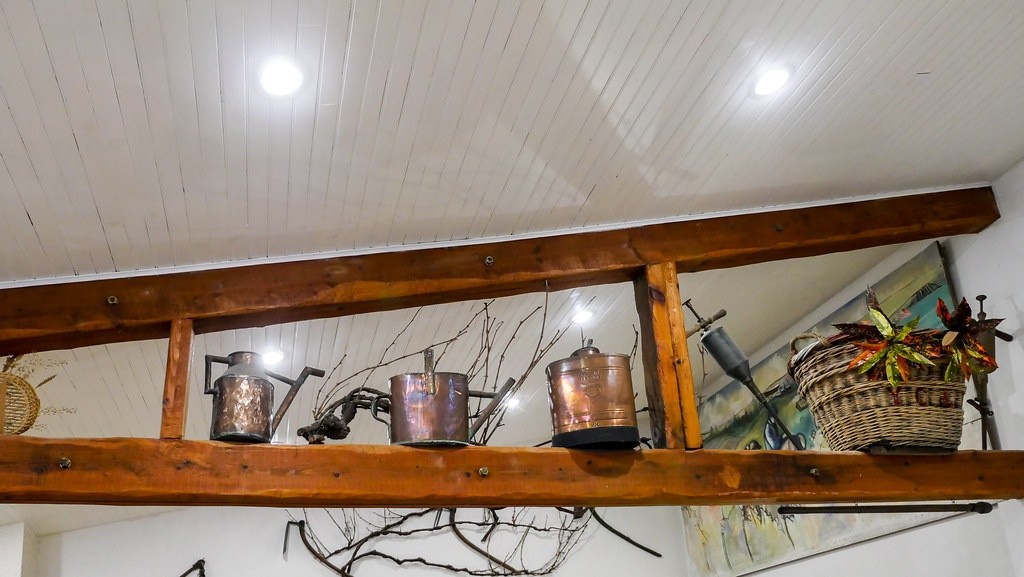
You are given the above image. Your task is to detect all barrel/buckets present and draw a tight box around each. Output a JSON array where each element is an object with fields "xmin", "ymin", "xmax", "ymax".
[
  {"xmin": 372, "ymin": 348, "xmax": 470, "ymax": 445},
  {"xmin": 544, "ymin": 347, "xmax": 640, "ymax": 448}
]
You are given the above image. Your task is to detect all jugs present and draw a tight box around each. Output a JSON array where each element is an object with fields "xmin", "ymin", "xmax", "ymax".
[{"xmin": 204, "ymin": 351, "xmax": 325, "ymax": 443}]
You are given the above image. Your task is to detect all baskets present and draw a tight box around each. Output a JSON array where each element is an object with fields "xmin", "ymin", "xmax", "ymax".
[
  {"xmin": 787, "ymin": 332, "xmax": 967, "ymax": 450},
  {"xmin": 0, "ymin": 372, "xmax": 40, "ymax": 435}
]
